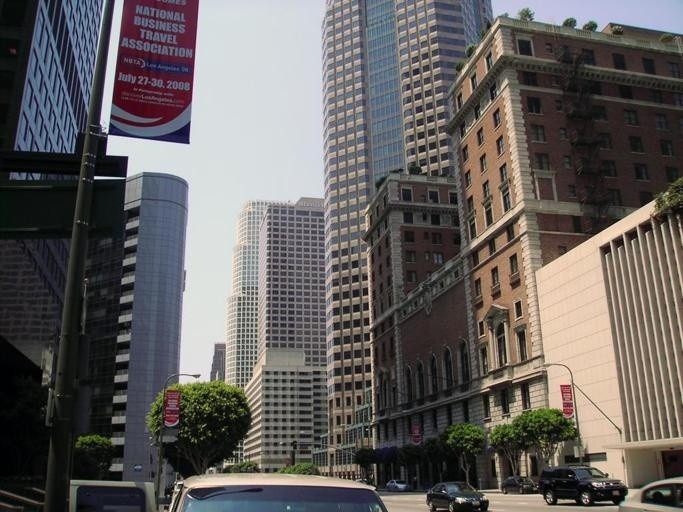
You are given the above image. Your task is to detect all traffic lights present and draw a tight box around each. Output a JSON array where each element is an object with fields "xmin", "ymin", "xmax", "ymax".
[{"xmin": 293, "ymin": 440, "xmax": 297, "ymax": 449}]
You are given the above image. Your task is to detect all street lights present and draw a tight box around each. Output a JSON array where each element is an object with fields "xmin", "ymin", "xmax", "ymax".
[
  {"xmin": 543, "ymin": 362, "xmax": 582, "ymax": 463},
  {"xmin": 341, "ymin": 423, "xmax": 358, "ymax": 451},
  {"xmin": 155, "ymin": 373, "xmax": 201, "ymax": 512}
]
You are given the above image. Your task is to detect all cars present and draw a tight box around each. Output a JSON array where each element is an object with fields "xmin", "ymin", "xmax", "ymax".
[
  {"xmin": 386, "ymin": 479, "xmax": 412, "ymax": 492},
  {"xmin": 426, "ymin": 481, "xmax": 489, "ymax": 512},
  {"xmin": 501, "ymin": 463, "xmax": 683, "ymax": 512}
]
